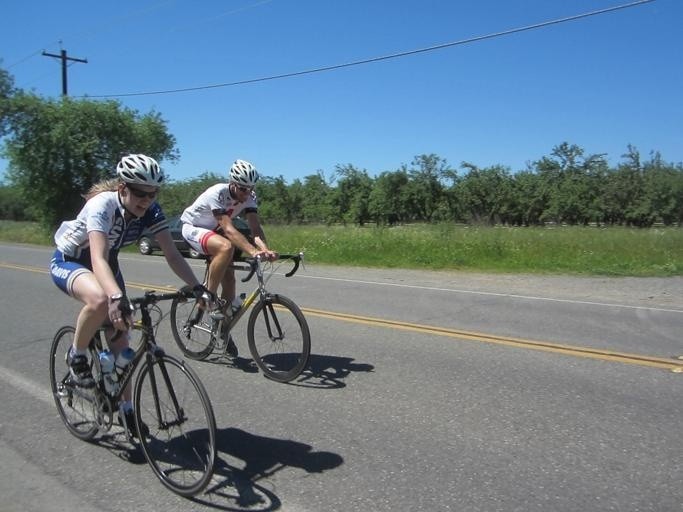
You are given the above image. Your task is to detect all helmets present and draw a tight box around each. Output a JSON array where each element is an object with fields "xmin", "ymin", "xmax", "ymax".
[
  {"xmin": 229, "ymin": 159, "xmax": 259, "ymax": 187},
  {"xmin": 116, "ymin": 153, "xmax": 165, "ymax": 188}
]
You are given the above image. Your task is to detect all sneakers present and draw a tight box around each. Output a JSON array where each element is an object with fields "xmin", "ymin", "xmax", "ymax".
[
  {"xmin": 118, "ymin": 407, "xmax": 150, "ymax": 439},
  {"xmin": 64, "ymin": 343, "xmax": 97, "ymax": 390},
  {"xmin": 222, "ymin": 334, "xmax": 239, "ymax": 360},
  {"xmin": 208, "ymin": 304, "xmax": 225, "ymax": 321}
]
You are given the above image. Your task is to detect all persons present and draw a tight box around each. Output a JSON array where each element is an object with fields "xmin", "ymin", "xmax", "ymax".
[
  {"xmin": 179, "ymin": 159, "xmax": 279, "ymax": 358},
  {"xmin": 52, "ymin": 152, "xmax": 206, "ymax": 438}
]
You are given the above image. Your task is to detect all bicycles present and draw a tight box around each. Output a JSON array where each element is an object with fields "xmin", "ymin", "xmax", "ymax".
[
  {"xmin": 164, "ymin": 247, "xmax": 313, "ymax": 383},
  {"xmin": 50, "ymin": 285, "xmax": 227, "ymax": 497}
]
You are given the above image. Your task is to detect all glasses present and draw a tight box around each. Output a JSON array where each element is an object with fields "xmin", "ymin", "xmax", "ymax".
[
  {"xmin": 234, "ymin": 182, "xmax": 254, "ymax": 192},
  {"xmin": 126, "ymin": 183, "xmax": 157, "ymax": 199}
]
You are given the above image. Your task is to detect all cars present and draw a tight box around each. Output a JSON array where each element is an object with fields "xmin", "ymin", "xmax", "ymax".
[{"xmin": 137, "ymin": 214, "xmax": 253, "ymax": 259}]
